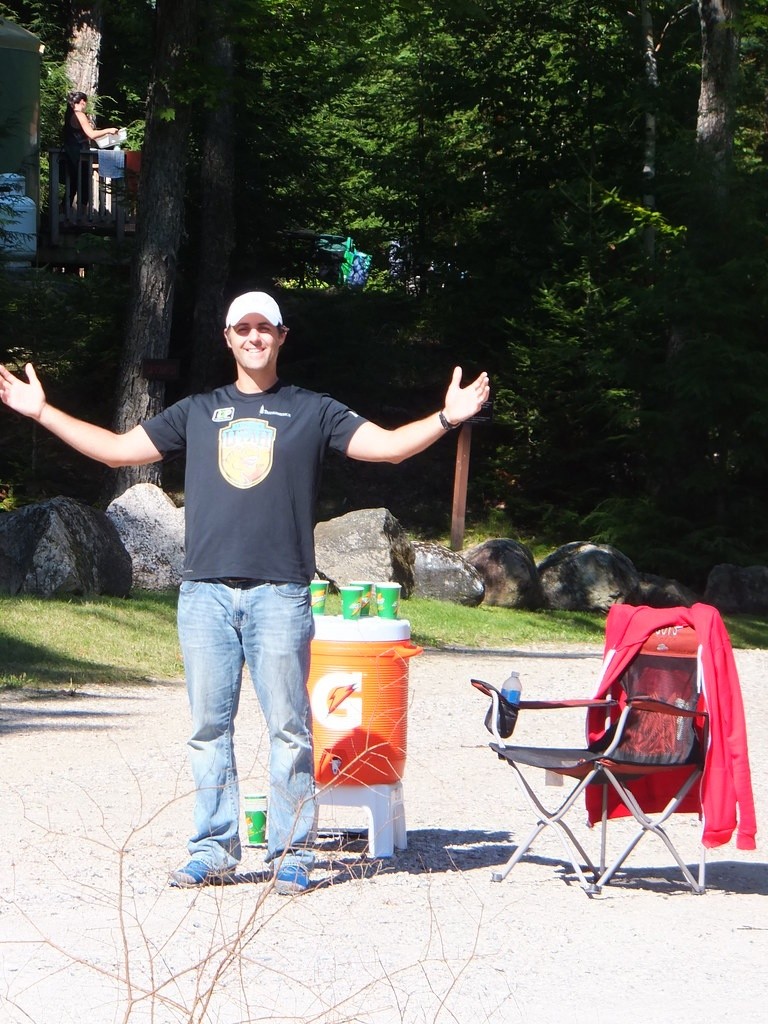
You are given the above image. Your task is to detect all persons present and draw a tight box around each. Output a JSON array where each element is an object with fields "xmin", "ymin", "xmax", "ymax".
[
  {"xmin": 0, "ymin": 291, "xmax": 491, "ymax": 894},
  {"xmin": 60, "ymin": 89, "xmax": 119, "ymax": 219}
]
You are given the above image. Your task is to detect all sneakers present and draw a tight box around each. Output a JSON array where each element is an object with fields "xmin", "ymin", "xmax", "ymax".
[
  {"xmin": 273, "ymin": 865, "xmax": 310, "ymax": 895},
  {"xmin": 167, "ymin": 860, "xmax": 237, "ymax": 888}
]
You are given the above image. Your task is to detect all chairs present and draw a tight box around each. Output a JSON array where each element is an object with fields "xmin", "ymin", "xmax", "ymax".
[{"xmin": 470, "ymin": 603, "xmax": 720, "ymax": 895}]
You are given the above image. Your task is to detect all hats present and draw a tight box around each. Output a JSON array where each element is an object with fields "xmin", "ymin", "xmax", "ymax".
[{"xmin": 226, "ymin": 291, "xmax": 283, "ymax": 329}]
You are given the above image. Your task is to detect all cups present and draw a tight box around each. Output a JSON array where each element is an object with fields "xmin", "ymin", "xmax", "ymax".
[
  {"xmin": 309, "ymin": 579, "xmax": 329, "ymax": 615},
  {"xmin": 376, "ymin": 583, "xmax": 403, "ymax": 620},
  {"xmin": 242, "ymin": 794, "xmax": 268, "ymax": 844},
  {"xmin": 351, "ymin": 581, "xmax": 373, "ymax": 618},
  {"xmin": 341, "ymin": 586, "xmax": 364, "ymax": 620}
]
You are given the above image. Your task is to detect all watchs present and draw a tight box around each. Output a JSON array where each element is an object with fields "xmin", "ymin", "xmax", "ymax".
[{"xmin": 437, "ymin": 406, "xmax": 462, "ymax": 432}]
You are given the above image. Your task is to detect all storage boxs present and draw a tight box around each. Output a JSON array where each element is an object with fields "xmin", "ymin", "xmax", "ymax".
[{"xmin": 302, "ymin": 616, "xmax": 425, "ymax": 784}]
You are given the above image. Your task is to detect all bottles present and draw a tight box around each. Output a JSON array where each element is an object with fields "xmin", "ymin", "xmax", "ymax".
[{"xmin": 501, "ymin": 670, "xmax": 521, "ymax": 706}]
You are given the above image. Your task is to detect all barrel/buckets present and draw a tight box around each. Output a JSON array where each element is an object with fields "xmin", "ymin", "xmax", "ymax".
[{"xmin": 305, "ymin": 614, "xmax": 421, "ymax": 786}]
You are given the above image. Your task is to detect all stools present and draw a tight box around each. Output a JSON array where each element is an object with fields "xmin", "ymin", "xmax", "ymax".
[{"xmin": 305, "ymin": 782, "xmax": 409, "ymax": 858}]
